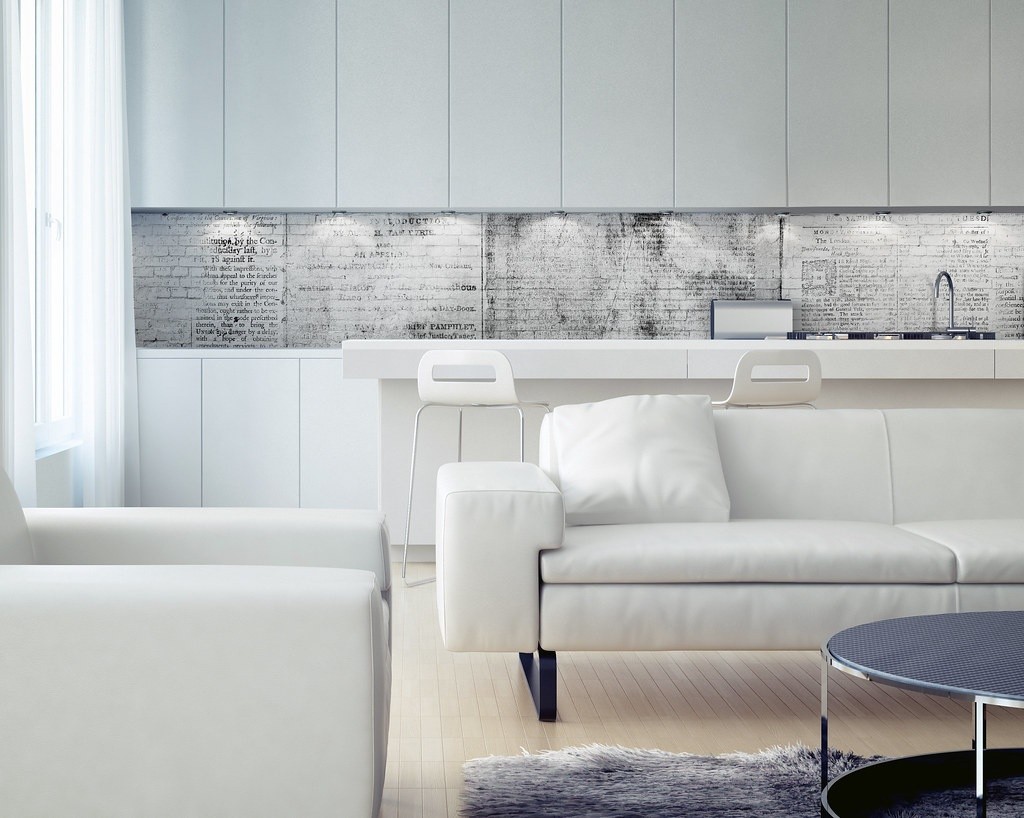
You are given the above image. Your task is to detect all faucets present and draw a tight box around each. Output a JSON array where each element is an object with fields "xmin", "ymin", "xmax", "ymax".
[{"xmin": 934, "ymin": 271, "xmax": 976, "ymax": 339}]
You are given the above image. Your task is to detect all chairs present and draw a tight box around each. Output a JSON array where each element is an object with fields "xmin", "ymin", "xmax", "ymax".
[{"xmin": 0, "ymin": 485, "xmax": 391, "ymax": 818}]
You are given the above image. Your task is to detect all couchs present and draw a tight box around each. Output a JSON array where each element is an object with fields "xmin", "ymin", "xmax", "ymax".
[{"xmin": 437, "ymin": 409, "xmax": 1023, "ymax": 722}]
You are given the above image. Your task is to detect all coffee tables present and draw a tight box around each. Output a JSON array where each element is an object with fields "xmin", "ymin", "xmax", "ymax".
[{"xmin": 821, "ymin": 611, "xmax": 1024, "ymax": 818}]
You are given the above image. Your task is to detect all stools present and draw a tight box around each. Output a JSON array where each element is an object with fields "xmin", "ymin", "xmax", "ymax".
[
  {"xmin": 713, "ymin": 350, "xmax": 822, "ymax": 410},
  {"xmin": 399, "ymin": 350, "xmax": 550, "ymax": 587}
]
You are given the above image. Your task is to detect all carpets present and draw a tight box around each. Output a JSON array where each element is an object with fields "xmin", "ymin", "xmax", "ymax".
[{"xmin": 458, "ymin": 745, "xmax": 888, "ymax": 818}]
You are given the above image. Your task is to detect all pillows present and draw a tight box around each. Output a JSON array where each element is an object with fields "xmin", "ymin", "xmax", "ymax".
[{"xmin": 552, "ymin": 395, "xmax": 728, "ymax": 522}]
